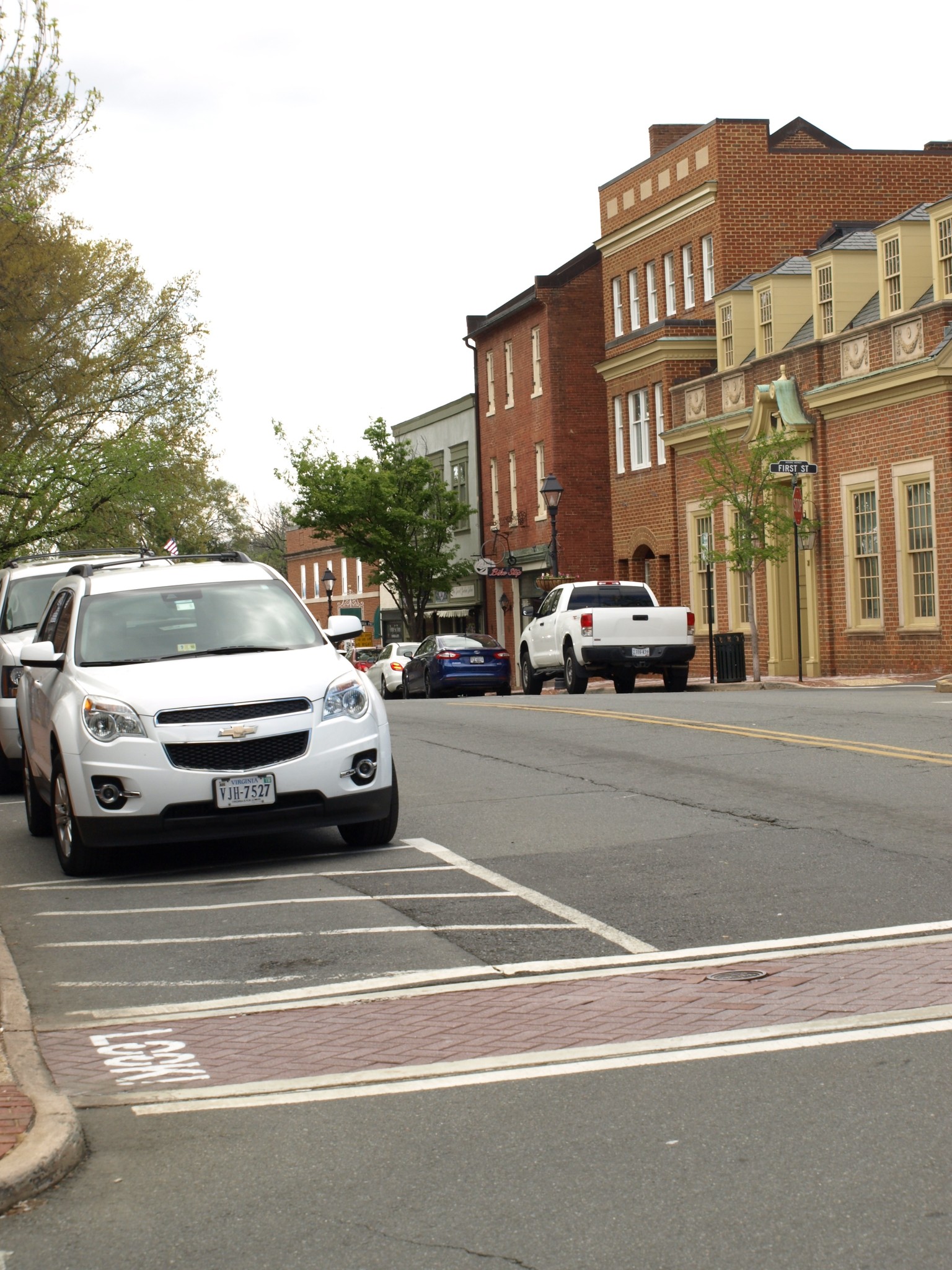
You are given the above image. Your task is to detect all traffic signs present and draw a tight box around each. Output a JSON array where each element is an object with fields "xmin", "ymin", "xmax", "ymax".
[
  {"xmin": 360, "ymin": 621, "xmax": 374, "ymax": 626},
  {"xmin": 770, "ymin": 460, "xmax": 817, "ymax": 474}
]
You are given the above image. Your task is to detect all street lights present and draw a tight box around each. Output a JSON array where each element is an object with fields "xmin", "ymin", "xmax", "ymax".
[
  {"xmin": 321, "ymin": 568, "xmax": 337, "ymax": 617},
  {"xmin": 539, "ymin": 473, "xmax": 563, "ymax": 576}
]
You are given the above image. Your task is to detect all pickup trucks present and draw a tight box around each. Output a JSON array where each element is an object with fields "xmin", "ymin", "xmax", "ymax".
[{"xmin": 518, "ymin": 581, "xmax": 697, "ymax": 695}]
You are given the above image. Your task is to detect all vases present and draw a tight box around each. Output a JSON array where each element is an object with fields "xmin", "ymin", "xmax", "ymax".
[{"xmin": 537, "ymin": 578, "xmax": 578, "ymax": 591}]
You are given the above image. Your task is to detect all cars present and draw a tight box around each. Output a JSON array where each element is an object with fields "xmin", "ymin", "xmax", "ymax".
[
  {"xmin": 365, "ymin": 642, "xmax": 422, "ymax": 700},
  {"xmin": 345, "ymin": 647, "xmax": 385, "ymax": 672},
  {"xmin": 402, "ymin": 633, "xmax": 512, "ymax": 699}
]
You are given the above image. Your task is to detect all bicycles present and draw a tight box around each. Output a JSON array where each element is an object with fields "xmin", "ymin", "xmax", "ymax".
[{"xmin": 480, "ymin": 526, "xmax": 518, "ymax": 567}]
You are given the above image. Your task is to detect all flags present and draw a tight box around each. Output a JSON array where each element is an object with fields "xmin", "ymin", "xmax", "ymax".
[{"xmin": 164, "ymin": 538, "xmax": 179, "ymax": 556}]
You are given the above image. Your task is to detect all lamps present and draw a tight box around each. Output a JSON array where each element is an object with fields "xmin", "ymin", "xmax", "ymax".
[
  {"xmin": 498, "ymin": 593, "xmax": 512, "ymax": 616},
  {"xmin": 796, "ymin": 500, "xmax": 821, "ymax": 551},
  {"xmin": 539, "ymin": 590, "xmax": 551, "ymax": 602}
]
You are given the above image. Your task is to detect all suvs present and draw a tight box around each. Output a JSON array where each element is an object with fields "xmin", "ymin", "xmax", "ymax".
[
  {"xmin": 15, "ymin": 550, "xmax": 399, "ymax": 878},
  {"xmin": 0, "ymin": 548, "xmax": 177, "ymax": 793}
]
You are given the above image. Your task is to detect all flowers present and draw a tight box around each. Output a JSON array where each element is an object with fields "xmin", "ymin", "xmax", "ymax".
[{"xmin": 538, "ymin": 570, "xmax": 582, "ymax": 581}]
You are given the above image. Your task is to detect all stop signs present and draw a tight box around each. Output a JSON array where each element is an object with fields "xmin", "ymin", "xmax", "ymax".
[{"xmin": 791, "ymin": 487, "xmax": 804, "ymax": 525}]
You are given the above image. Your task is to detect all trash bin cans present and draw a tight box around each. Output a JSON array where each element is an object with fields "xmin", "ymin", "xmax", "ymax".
[{"xmin": 713, "ymin": 632, "xmax": 747, "ymax": 684}]
